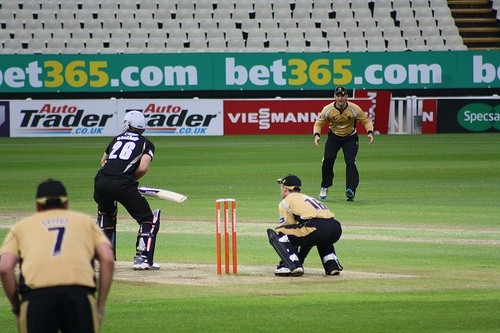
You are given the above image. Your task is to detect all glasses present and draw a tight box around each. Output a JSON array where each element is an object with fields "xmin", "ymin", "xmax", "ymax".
[{"xmin": 336, "ymin": 94, "xmax": 345, "ymax": 97}]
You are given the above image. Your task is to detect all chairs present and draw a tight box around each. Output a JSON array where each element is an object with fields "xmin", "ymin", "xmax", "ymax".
[{"xmin": 0, "ymin": 0, "xmax": 472, "ymax": 55}]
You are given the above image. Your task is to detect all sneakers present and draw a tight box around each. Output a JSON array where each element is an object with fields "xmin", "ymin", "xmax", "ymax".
[{"xmin": 133, "ymin": 255, "xmax": 161, "ymax": 270}]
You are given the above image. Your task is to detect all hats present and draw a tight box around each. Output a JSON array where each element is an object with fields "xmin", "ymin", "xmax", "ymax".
[
  {"xmin": 34, "ymin": 178, "xmax": 69, "ymax": 206},
  {"xmin": 335, "ymin": 86, "xmax": 348, "ymax": 94},
  {"xmin": 278, "ymin": 174, "xmax": 302, "ymax": 191}
]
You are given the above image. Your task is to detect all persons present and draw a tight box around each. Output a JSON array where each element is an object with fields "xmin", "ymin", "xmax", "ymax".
[
  {"xmin": 312, "ymin": 86, "xmax": 374, "ymax": 201},
  {"xmin": 93, "ymin": 111, "xmax": 161, "ymax": 270},
  {"xmin": 0, "ymin": 178, "xmax": 115, "ymax": 333},
  {"xmin": 266, "ymin": 175, "xmax": 342, "ymax": 276}
]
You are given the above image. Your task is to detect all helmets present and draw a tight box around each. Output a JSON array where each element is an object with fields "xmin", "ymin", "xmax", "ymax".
[{"xmin": 123, "ymin": 111, "xmax": 147, "ymax": 130}]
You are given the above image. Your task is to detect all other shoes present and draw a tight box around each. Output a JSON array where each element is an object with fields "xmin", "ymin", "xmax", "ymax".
[
  {"xmin": 345, "ymin": 188, "xmax": 355, "ymax": 201},
  {"xmin": 318, "ymin": 187, "xmax": 328, "ymax": 199},
  {"xmin": 327, "ymin": 269, "xmax": 340, "ymax": 275},
  {"xmin": 274, "ymin": 263, "xmax": 292, "ymax": 276}
]
